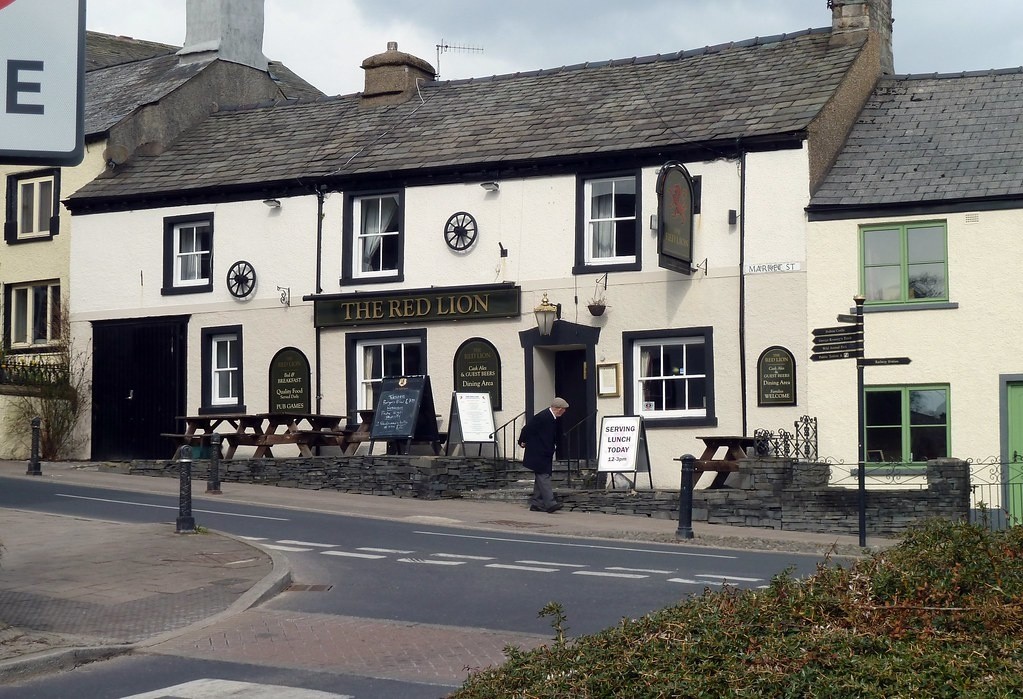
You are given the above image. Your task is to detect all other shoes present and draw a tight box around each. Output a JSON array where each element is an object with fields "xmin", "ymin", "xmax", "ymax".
[
  {"xmin": 530, "ymin": 505, "xmax": 543, "ymax": 511},
  {"xmin": 548, "ymin": 503, "xmax": 564, "ymax": 513}
]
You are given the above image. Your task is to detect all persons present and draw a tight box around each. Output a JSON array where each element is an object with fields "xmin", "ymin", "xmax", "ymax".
[{"xmin": 516, "ymin": 397, "xmax": 570, "ymax": 513}]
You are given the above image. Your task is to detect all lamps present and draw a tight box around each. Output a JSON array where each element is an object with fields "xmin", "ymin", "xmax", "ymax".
[
  {"xmin": 263, "ymin": 199, "xmax": 280, "ymax": 207},
  {"xmin": 481, "ymin": 181, "xmax": 500, "ymax": 192},
  {"xmin": 534, "ymin": 293, "xmax": 562, "ymax": 337}
]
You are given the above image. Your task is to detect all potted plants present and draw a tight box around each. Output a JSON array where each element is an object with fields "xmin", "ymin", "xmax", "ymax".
[{"xmin": 584, "ymin": 284, "xmax": 606, "ymax": 316}]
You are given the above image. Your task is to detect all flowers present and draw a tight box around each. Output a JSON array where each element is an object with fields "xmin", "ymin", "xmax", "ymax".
[{"xmin": 1, "ymin": 356, "xmax": 48, "ymax": 385}]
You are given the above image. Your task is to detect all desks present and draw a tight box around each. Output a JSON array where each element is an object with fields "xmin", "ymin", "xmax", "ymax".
[
  {"xmin": 333, "ymin": 410, "xmax": 376, "ymax": 457},
  {"xmin": 689, "ymin": 436, "xmax": 754, "ymax": 489},
  {"xmin": 251, "ymin": 412, "xmax": 351, "ymax": 459},
  {"xmin": 161, "ymin": 414, "xmax": 274, "ymax": 461}
]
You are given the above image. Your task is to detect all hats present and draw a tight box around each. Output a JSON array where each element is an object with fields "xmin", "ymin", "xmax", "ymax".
[{"xmin": 551, "ymin": 397, "xmax": 569, "ymax": 408}]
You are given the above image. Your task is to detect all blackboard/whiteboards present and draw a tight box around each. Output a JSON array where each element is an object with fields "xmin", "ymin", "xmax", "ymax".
[{"xmin": 367, "ymin": 373, "xmax": 439, "ymax": 455}]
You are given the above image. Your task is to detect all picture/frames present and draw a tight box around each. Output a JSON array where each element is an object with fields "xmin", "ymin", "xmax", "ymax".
[{"xmin": 597, "ymin": 363, "xmax": 620, "ymax": 398}]
